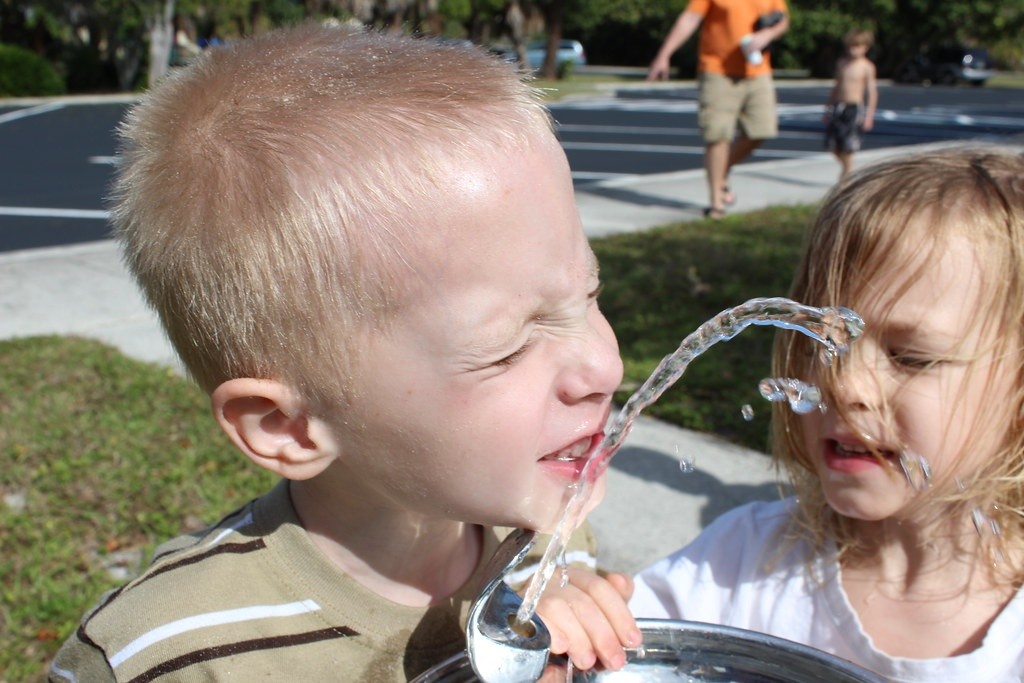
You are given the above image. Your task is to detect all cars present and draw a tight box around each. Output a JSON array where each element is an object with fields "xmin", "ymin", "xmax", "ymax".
[
  {"xmin": 502, "ymin": 38, "xmax": 588, "ymax": 70},
  {"xmin": 900, "ymin": 47, "xmax": 992, "ymax": 89}
]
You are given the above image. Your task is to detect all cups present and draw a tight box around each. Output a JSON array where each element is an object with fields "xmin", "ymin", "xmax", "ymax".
[{"xmin": 739, "ymin": 35, "xmax": 763, "ymax": 65}]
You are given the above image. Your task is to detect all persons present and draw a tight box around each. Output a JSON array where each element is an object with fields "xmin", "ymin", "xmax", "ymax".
[
  {"xmin": 647, "ymin": 0, "xmax": 791, "ymax": 220},
  {"xmin": 347, "ymin": 0, "xmax": 563, "ymax": 75},
  {"xmin": 40, "ymin": 18, "xmax": 625, "ymax": 683},
  {"xmin": 519, "ymin": 149, "xmax": 1024, "ymax": 683},
  {"xmin": 822, "ymin": 26, "xmax": 877, "ymax": 179}
]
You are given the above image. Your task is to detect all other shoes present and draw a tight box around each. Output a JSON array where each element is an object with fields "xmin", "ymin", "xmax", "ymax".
[
  {"xmin": 723, "ymin": 185, "xmax": 736, "ymax": 206},
  {"xmin": 704, "ymin": 206, "xmax": 725, "ymax": 220}
]
